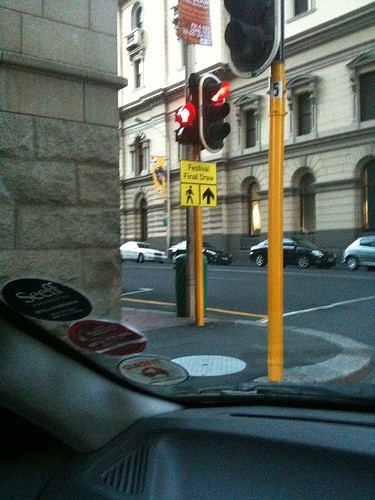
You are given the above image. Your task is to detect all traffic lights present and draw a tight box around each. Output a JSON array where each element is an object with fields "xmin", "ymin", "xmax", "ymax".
[{"xmin": 173, "ymin": 71, "xmax": 231, "ymax": 156}]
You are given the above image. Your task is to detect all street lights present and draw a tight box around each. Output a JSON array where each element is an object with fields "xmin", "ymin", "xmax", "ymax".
[{"xmin": 222, "ymin": 0, "xmax": 285, "ymax": 78}]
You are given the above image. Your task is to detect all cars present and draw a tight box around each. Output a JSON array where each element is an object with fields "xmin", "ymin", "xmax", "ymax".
[
  {"xmin": 249, "ymin": 236, "xmax": 337, "ymax": 268},
  {"xmin": 168, "ymin": 239, "xmax": 232, "ymax": 265},
  {"xmin": 342, "ymin": 235, "xmax": 375, "ymax": 269},
  {"xmin": 120, "ymin": 240, "xmax": 167, "ymax": 264}
]
